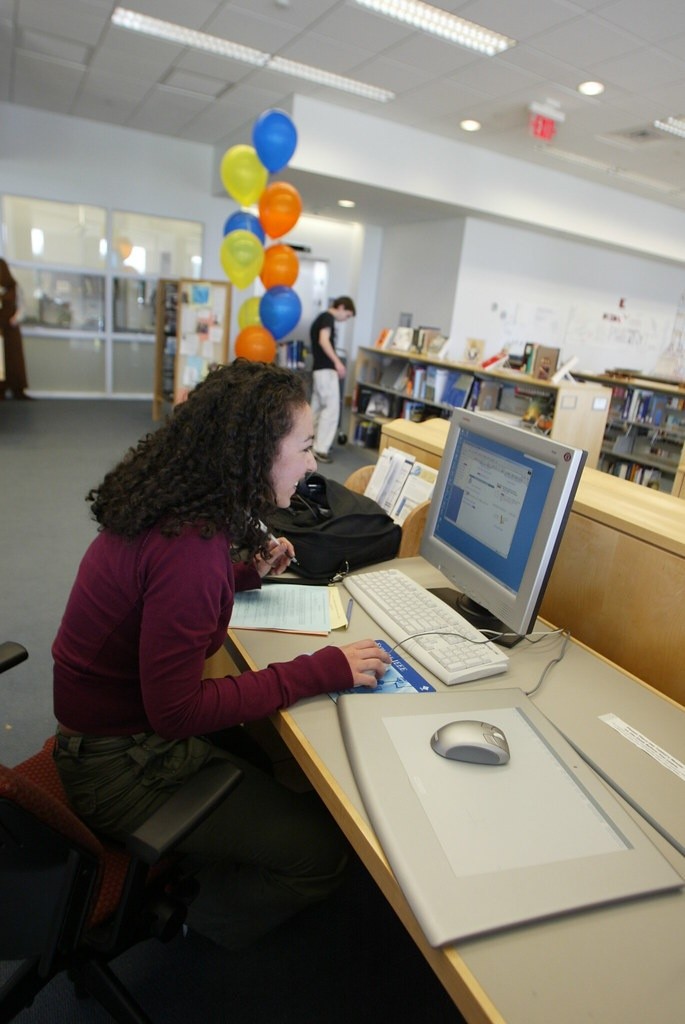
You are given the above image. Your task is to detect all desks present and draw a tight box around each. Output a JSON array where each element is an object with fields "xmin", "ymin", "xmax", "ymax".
[{"xmin": 223, "ymin": 418, "xmax": 685, "ymax": 1024}]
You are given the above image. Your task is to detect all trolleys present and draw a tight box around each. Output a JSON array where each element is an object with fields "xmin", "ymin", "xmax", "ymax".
[{"xmin": 301, "ymin": 347, "xmax": 348, "ymax": 446}]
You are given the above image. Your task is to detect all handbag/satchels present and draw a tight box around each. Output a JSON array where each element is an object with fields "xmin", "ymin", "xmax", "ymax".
[{"xmin": 260, "ymin": 472, "xmax": 403, "ymax": 587}]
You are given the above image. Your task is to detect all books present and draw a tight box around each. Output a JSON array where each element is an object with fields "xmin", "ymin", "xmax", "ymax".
[
  {"xmin": 599, "ymin": 383, "xmax": 685, "ymax": 493},
  {"xmin": 356, "ymin": 325, "xmax": 560, "ymax": 446}
]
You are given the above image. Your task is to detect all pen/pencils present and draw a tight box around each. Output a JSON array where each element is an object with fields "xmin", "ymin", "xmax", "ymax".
[
  {"xmin": 345, "ymin": 599, "xmax": 353, "ymax": 629},
  {"xmin": 267, "ymin": 530, "xmax": 300, "ymax": 565}
]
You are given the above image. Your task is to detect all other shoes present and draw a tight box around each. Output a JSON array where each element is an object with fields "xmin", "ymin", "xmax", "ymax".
[
  {"xmin": 11, "ymin": 391, "xmax": 33, "ymax": 401},
  {"xmin": 313, "ymin": 452, "xmax": 332, "ymax": 464}
]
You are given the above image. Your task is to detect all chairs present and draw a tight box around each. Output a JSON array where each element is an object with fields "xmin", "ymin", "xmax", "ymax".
[{"xmin": 0, "ymin": 638, "xmax": 243, "ymax": 1024}]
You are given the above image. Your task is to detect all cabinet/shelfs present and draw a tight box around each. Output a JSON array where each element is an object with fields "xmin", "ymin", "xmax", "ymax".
[
  {"xmin": 345, "ymin": 344, "xmax": 685, "ymax": 499},
  {"xmin": 152, "ymin": 277, "xmax": 233, "ymax": 422}
]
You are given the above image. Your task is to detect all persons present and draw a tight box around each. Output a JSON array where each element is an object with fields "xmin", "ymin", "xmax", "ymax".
[
  {"xmin": 309, "ymin": 296, "xmax": 356, "ymax": 464},
  {"xmin": 0, "ymin": 259, "xmax": 33, "ymax": 401},
  {"xmin": 51, "ymin": 357, "xmax": 393, "ymax": 953}
]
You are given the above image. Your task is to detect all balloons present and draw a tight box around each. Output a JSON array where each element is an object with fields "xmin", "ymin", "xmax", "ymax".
[{"xmin": 221, "ymin": 111, "xmax": 303, "ymax": 367}]
